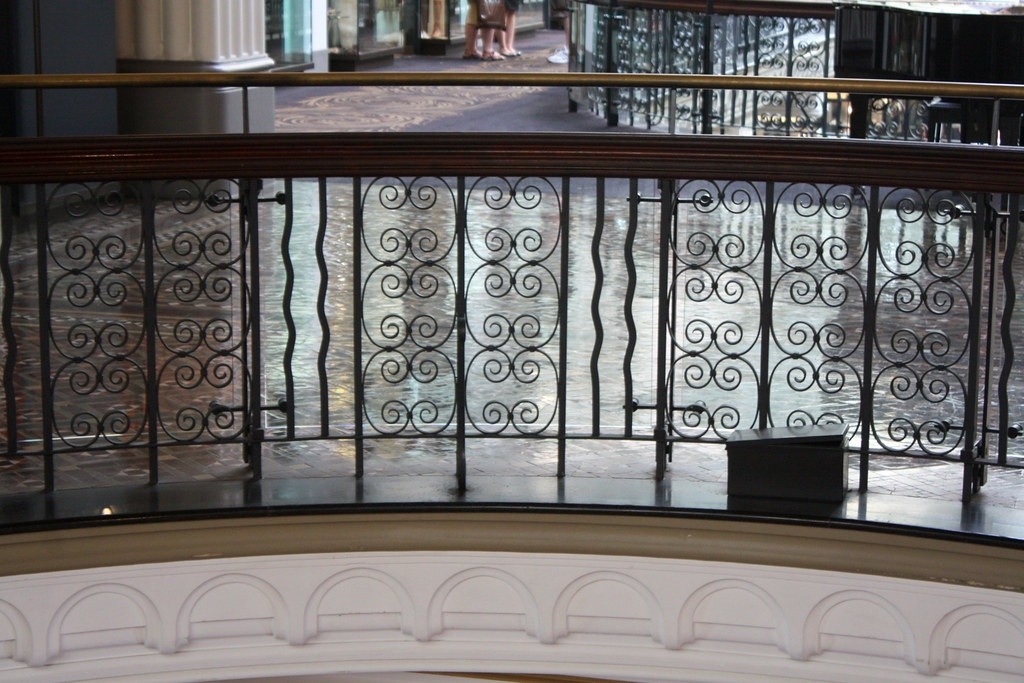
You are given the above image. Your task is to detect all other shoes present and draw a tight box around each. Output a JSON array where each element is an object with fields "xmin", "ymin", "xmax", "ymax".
[
  {"xmin": 508, "ymin": 47, "xmax": 521, "ymax": 55},
  {"xmin": 548, "ymin": 45, "xmax": 581, "ymax": 63},
  {"xmin": 463, "ymin": 48, "xmax": 483, "ymax": 58},
  {"xmin": 482, "ymin": 49, "xmax": 506, "ymax": 60},
  {"xmin": 500, "ymin": 49, "xmax": 516, "ymax": 57}
]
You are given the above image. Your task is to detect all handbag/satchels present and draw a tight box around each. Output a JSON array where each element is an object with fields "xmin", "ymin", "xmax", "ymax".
[{"xmin": 476, "ymin": 0, "xmax": 506, "ymax": 30}]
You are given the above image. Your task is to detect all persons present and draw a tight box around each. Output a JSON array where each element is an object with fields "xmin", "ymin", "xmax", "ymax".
[
  {"xmin": 545, "ymin": 0, "xmax": 571, "ymax": 64},
  {"xmin": 421, "ymin": 0, "xmax": 443, "ymax": 38},
  {"xmin": 463, "ymin": 0, "xmax": 524, "ymax": 61}
]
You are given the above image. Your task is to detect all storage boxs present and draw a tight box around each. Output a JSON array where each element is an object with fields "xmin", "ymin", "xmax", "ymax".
[{"xmin": 725, "ymin": 423, "xmax": 848, "ymax": 502}]
